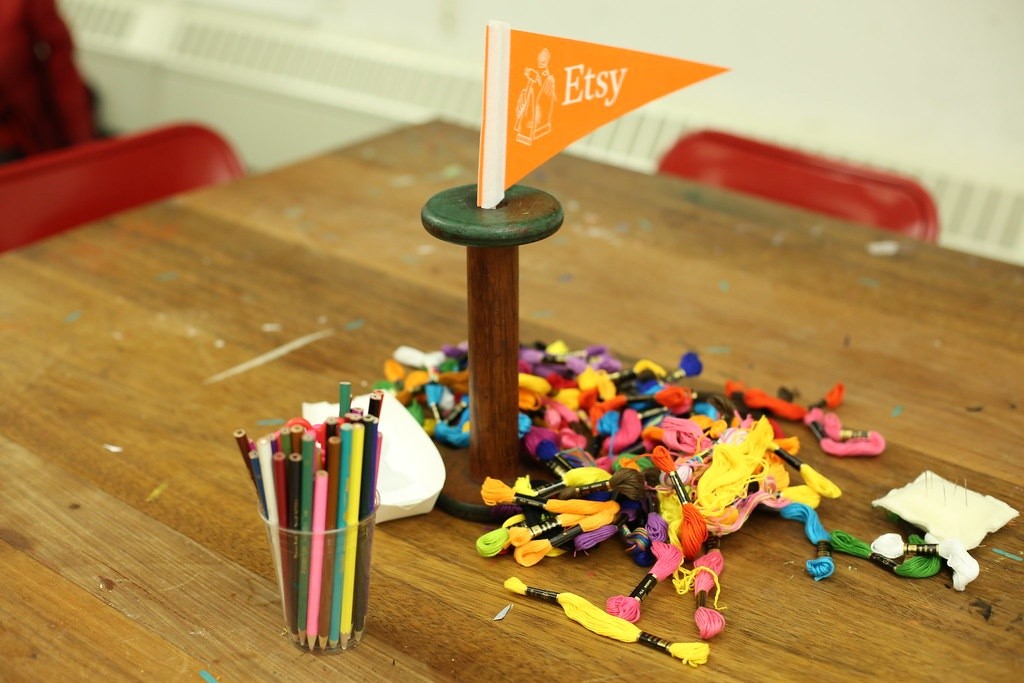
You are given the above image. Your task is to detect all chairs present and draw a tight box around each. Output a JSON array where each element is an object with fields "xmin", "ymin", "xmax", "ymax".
[
  {"xmin": 0, "ymin": 124, "xmax": 244, "ymax": 256},
  {"xmin": 659, "ymin": 130, "xmax": 938, "ymax": 240}
]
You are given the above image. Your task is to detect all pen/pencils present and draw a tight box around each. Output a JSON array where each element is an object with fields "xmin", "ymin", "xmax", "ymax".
[{"xmin": 234, "ymin": 381, "xmax": 384, "ymax": 653}]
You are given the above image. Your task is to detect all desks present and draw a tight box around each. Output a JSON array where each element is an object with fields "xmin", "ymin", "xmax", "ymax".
[{"xmin": 0, "ymin": 116, "xmax": 1024, "ymax": 683}]
